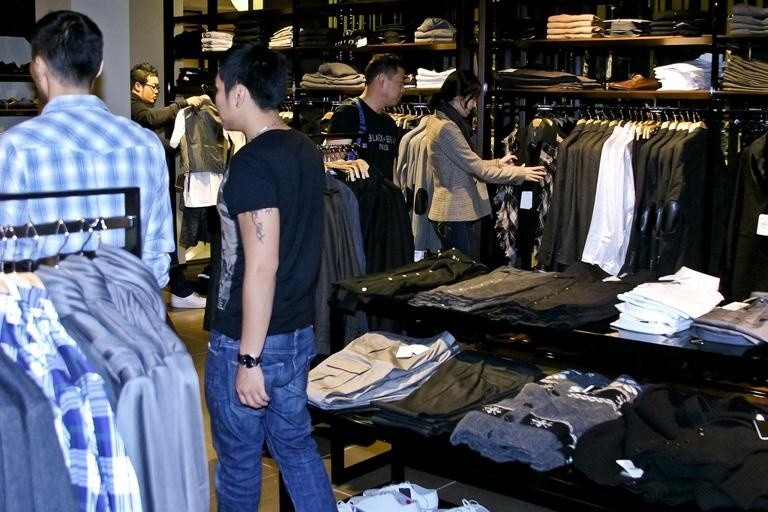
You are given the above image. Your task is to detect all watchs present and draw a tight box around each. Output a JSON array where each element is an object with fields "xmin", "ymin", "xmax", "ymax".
[{"xmin": 235, "ymin": 352, "xmax": 264, "ymax": 367}]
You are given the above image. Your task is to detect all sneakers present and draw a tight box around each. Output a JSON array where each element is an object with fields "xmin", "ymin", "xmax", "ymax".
[
  {"xmin": 336, "ymin": 481, "xmax": 439, "ymax": 512},
  {"xmin": 438, "ymin": 498, "xmax": 490, "ymax": 512},
  {"xmin": 170, "ymin": 292, "xmax": 207, "ymax": 309},
  {"xmin": 197, "ymin": 264, "xmax": 211, "ymax": 279}
]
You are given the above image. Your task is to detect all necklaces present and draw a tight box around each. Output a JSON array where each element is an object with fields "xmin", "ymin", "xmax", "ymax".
[{"xmin": 246, "ymin": 116, "xmax": 286, "ymax": 143}]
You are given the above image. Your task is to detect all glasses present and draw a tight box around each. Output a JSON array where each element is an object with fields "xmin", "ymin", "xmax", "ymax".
[{"xmin": 143, "ymin": 83, "xmax": 160, "ymax": 92}]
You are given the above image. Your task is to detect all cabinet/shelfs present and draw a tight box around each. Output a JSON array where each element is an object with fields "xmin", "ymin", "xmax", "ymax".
[
  {"xmin": 484, "ymin": 2, "xmax": 767, "ymax": 275},
  {"xmin": 303, "ymin": 284, "xmax": 768, "ymax": 511},
  {"xmin": 163, "ymin": 1, "xmax": 483, "ymax": 275}
]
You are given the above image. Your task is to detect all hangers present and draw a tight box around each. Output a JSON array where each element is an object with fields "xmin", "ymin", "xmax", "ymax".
[
  {"xmin": 0, "ymin": 216, "xmax": 135, "ymax": 303},
  {"xmin": 278, "ymin": 94, "xmax": 435, "ymax": 126},
  {"xmin": 507, "ymin": 100, "xmax": 713, "ymax": 132}
]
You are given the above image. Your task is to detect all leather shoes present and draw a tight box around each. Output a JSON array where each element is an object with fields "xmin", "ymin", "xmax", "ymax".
[
  {"xmin": 608, "ymin": 73, "xmax": 661, "ymax": 90},
  {"xmin": 0, "ymin": 61, "xmax": 32, "ymax": 75}
]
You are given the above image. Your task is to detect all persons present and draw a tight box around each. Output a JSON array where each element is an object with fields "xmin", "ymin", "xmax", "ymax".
[
  {"xmin": 422, "ymin": 70, "xmax": 549, "ymax": 349},
  {"xmin": 0, "ymin": 9, "xmax": 175, "ymax": 293},
  {"xmin": 131, "ymin": 61, "xmax": 209, "ymax": 314},
  {"xmin": 324, "ymin": 52, "xmax": 407, "ymax": 183},
  {"xmin": 198, "ymin": 40, "xmax": 337, "ymax": 511}
]
